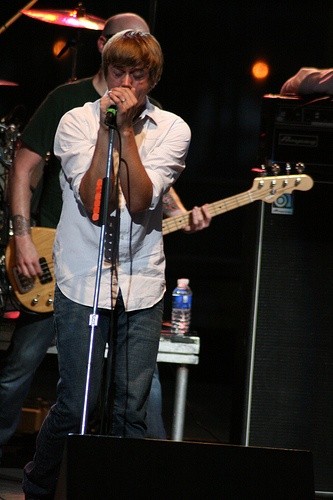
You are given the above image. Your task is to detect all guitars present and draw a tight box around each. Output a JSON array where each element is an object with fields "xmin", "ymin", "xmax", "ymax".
[{"xmin": 5, "ymin": 161, "xmax": 314, "ymax": 316}]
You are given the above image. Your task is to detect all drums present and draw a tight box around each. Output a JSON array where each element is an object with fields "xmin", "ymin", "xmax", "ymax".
[{"xmin": 0, "ymin": 118, "xmax": 50, "ymax": 201}]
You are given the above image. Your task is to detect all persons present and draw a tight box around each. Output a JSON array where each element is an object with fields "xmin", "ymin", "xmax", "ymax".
[
  {"xmin": 0, "ymin": 12, "xmax": 211, "ymax": 458},
  {"xmin": 22, "ymin": 29, "xmax": 191, "ymax": 500}
]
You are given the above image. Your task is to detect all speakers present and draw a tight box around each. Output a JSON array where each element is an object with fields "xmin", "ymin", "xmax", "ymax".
[
  {"xmin": 244, "ymin": 180, "xmax": 333, "ymax": 500},
  {"xmin": 65, "ymin": 433, "xmax": 316, "ymax": 500}
]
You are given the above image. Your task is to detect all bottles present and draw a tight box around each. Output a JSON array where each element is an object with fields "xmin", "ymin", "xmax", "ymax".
[{"xmin": 171, "ymin": 279, "xmax": 192, "ymax": 338}]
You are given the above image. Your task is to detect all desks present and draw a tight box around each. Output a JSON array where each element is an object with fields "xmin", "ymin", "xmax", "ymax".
[{"xmin": 0, "ymin": 324, "xmax": 201, "ymax": 442}]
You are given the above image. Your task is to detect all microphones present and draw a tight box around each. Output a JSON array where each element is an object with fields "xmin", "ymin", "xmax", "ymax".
[{"xmin": 104, "ymin": 105, "xmax": 118, "ymax": 124}]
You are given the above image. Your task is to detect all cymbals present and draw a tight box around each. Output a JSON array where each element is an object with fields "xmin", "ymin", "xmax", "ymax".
[
  {"xmin": 0, "ymin": 79, "xmax": 19, "ymax": 86},
  {"xmin": 20, "ymin": 9, "xmax": 108, "ymax": 30}
]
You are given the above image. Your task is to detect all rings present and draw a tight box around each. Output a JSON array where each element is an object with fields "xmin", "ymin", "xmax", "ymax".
[{"xmin": 121, "ymin": 99, "xmax": 127, "ymax": 103}]
